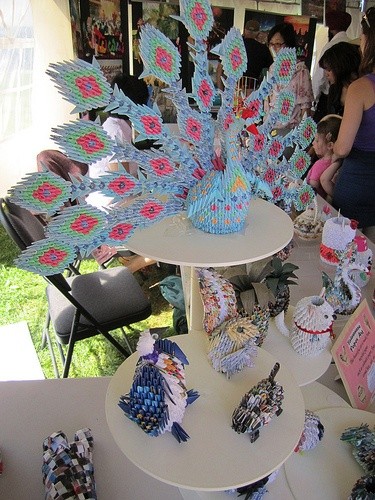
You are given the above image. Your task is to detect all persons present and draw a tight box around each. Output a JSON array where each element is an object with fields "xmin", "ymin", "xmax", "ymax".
[
  {"xmin": 38, "ymin": 8, "xmax": 374, "ymax": 221},
  {"xmin": 333, "ymin": 6, "xmax": 375, "ymax": 235}
]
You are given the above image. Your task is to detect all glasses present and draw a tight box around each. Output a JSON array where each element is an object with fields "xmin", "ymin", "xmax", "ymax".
[{"xmin": 266, "ymin": 41, "xmax": 285, "ymax": 48}]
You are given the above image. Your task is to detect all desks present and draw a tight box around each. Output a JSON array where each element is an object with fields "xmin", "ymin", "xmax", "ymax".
[{"xmin": 0, "ymin": 196, "xmax": 375, "ymax": 500}]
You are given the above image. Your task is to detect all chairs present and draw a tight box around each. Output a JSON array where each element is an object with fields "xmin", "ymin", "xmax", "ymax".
[{"xmin": 0, "ymin": 196, "xmax": 153, "ymax": 378}]
